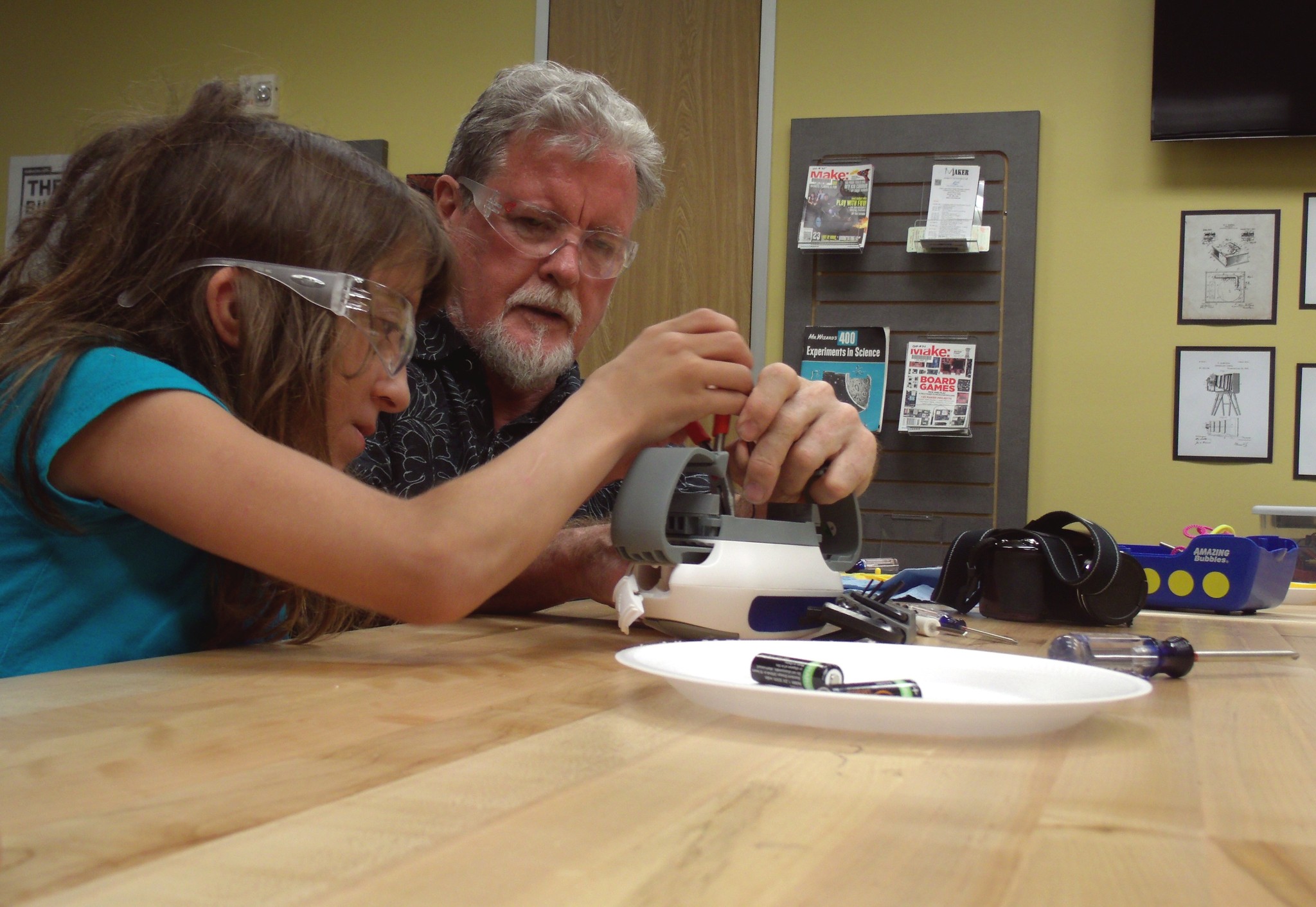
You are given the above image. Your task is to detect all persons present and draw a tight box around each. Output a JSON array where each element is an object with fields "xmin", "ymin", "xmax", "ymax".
[
  {"xmin": 342, "ymin": 63, "xmax": 878, "ymax": 612},
  {"xmin": 0, "ymin": 81, "xmax": 751, "ymax": 679}
]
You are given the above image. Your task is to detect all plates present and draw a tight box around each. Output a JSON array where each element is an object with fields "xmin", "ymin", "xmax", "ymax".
[{"xmin": 614, "ymin": 638, "xmax": 1152, "ymax": 739}]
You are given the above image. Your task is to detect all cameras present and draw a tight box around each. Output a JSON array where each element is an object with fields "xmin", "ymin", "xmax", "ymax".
[{"xmin": 979, "ymin": 530, "xmax": 1148, "ymax": 628}]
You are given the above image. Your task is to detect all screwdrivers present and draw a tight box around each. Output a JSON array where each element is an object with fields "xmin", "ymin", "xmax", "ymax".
[
  {"xmin": 1045, "ymin": 629, "xmax": 1302, "ymax": 683},
  {"xmin": 906, "ymin": 601, "xmax": 1020, "ymax": 645},
  {"xmin": 711, "ymin": 411, "xmax": 732, "ymax": 453}
]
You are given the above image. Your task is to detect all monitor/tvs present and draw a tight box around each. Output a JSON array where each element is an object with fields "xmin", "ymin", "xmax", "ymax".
[{"xmin": 1150, "ymin": 1, "xmax": 1316, "ymax": 142}]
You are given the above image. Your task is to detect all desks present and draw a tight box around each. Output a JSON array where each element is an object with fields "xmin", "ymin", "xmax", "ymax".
[{"xmin": 3, "ymin": 572, "xmax": 1316, "ymax": 905}]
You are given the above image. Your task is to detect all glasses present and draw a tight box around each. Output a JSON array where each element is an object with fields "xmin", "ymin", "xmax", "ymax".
[
  {"xmin": 455, "ymin": 174, "xmax": 637, "ymax": 282},
  {"xmin": 116, "ymin": 256, "xmax": 420, "ymax": 380}
]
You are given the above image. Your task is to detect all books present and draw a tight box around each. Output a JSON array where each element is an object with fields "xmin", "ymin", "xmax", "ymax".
[
  {"xmin": 797, "ymin": 164, "xmax": 874, "ymax": 248},
  {"xmin": 799, "ymin": 326, "xmax": 891, "ymax": 433},
  {"xmin": 898, "ymin": 342, "xmax": 976, "ymax": 435}
]
